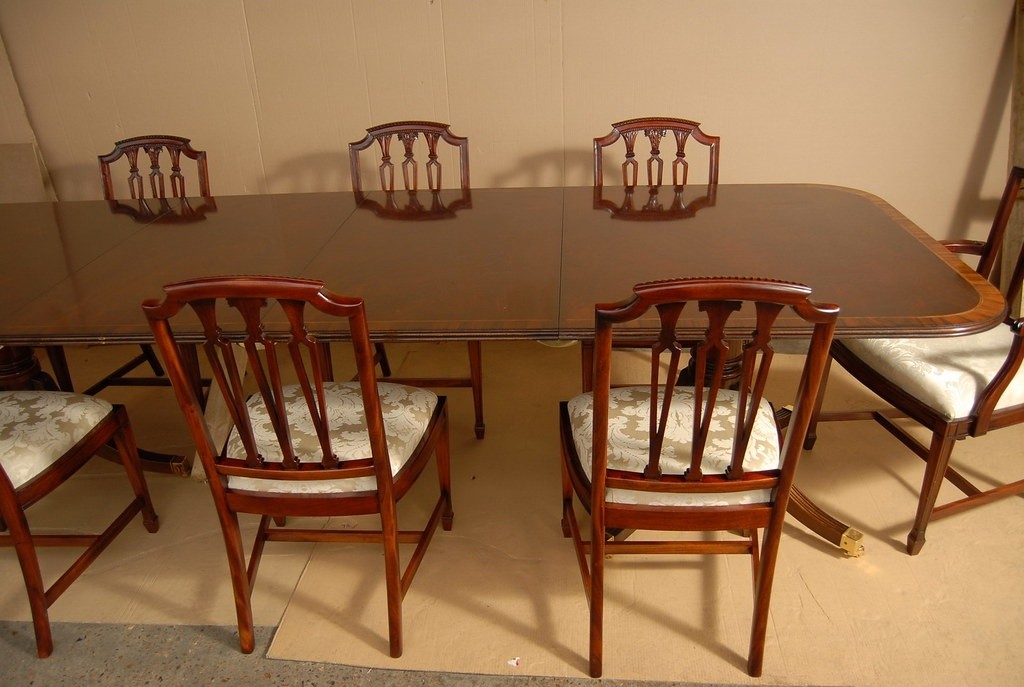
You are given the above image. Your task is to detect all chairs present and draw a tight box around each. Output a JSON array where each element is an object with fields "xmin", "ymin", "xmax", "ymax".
[
  {"xmin": 138, "ymin": 273, "xmax": 456, "ymax": 658},
  {"xmin": 94, "ymin": 117, "xmax": 724, "ymax": 443},
  {"xmin": 801, "ymin": 164, "xmax": 1024, "ymax": 556},
  {"xmin": 0, "ymin": 388, "xmax": 160, "ymax": 660},
  {"xmin": 552, "ymin": 278, "xmax": 842, "ymax": 680}
]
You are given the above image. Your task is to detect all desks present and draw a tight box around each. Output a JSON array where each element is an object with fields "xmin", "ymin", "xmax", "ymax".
[{"xmin": 0, "ymin": 181, "xmax": 1010, "ymax": 557}]
